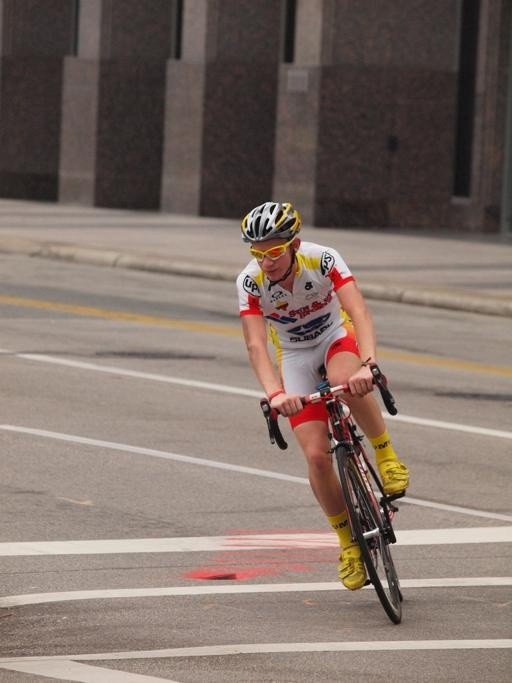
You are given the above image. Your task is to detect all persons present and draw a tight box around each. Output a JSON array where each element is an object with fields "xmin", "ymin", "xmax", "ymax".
[{"xmin": 233, "ymin": 198, "xmax": 410, "ymax": 594}]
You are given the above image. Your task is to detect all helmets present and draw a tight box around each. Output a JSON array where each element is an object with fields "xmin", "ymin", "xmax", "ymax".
[{"xmin": 241, "ymin": 201, "xmax": 301, "ymax": 242}]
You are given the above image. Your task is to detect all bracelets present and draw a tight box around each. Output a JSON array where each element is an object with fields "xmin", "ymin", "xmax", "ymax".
[
  {"xmin": 266, "ymin": 389, "xmax": 286, "ymax": 402},
  {"xmin": 360, "ymin": 360, "xmax": 378, "ymax": 367}
]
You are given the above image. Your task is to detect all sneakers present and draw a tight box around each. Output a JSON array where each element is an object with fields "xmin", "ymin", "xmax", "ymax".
[
  {"xmin": 377, "ymin": 458, "xmax": 409, "ymax": 497},
  {"xmin": 338, "ymin": 538, "xmax": 367, "ymax": 592}
]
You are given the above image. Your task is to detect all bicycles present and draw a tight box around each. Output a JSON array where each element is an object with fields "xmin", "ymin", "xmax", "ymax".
[{"xmin": 254, "ymin": 358, "xmax": 413, "ymax": 626}]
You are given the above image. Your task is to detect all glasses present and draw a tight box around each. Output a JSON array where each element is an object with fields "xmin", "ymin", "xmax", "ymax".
[{"xmin": 249, "ymin": 235, "xmax": 297, "ymax": 262}]
